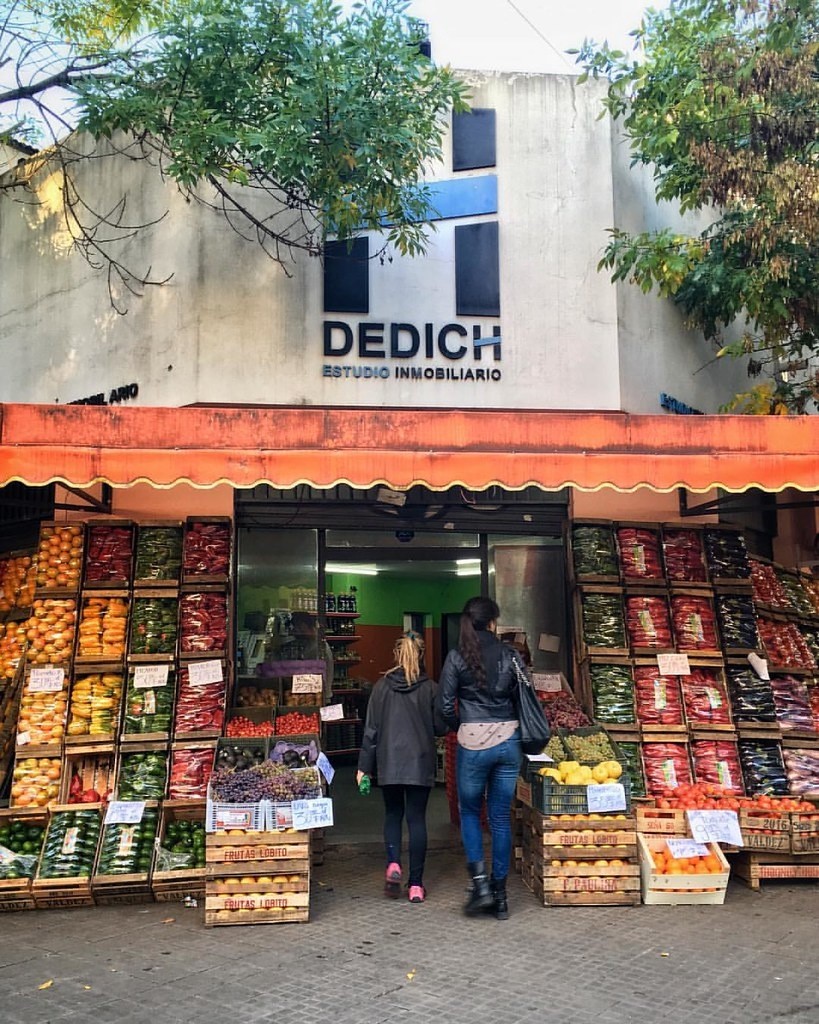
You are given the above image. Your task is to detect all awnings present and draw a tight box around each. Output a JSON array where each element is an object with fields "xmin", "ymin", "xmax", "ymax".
[{"xmin": 0, "ymin": 403, "xmax": 819, "ymax": 492}]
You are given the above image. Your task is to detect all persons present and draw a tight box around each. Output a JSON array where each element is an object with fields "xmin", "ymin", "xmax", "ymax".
[
  {"xmin": 437, "ymin": 596, "xmax": 524, "ymax": 921},
  {"xmin": 278, "ymin": 611, "xmax": 334, "ymax": 705},
  {"xmin": 357, "ymin": 631, "xmax": 451, "ymax": 903}
]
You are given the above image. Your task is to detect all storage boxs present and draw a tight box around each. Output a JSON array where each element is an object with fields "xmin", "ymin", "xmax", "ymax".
[
  {"xmin": 151, "ymin": 798, "xmax": 205, "ymax": 902},
  {"xmin": 265, "ymin": 732, "xmax": 322, "ymax": 831},
  {"xmin": 738, "ymin": 806, "xmax": 791, "ymax": 853},
  {"xmin": 204, "ymin": 875, "xmax": 310, "ymax": 930},
  {"xmin": 91, "ymin": 801, "xmax": 162, "ymax": 903},
  {"xmin": 520, "ymin": 842, "xmax": 642, "ymax": 907},
  {"xmin": 556, "ymin": 724, "xmax": 627, "ymax": 774},
  {"xmin": 731, "ymin": 853, "xmax": 819, "ymax": 892},
  {"xmin": 204, "ymin": 830, "xmax": 310, "ymax": 875},
  {"xmin": 31, "ymin": 802, "xmax": 106, "ymax": 911},
  {"xmin": 791, "ymin": 810, "xmax": 818, "ymax": 855},
  {"xmin": 528, "ymin": 769, "xmax": 632, "ymax": 816},
  {"xmin": 0, "ymin": 807, "xmax": 50, "ymax": 912},
  {"xmin": 0, "ymin": 516, "xmax": 327, "ymax": 808},
  {"xmin": 563, "ymin": 516, "xmax": 819, "ymax": 805},
  {"xmin": 636, "ymin": 832, "xmax": 731, "ymax": 906},
  {"xmin": 520, "ymin": 804, "xmax": 642, "ymax": 859}
]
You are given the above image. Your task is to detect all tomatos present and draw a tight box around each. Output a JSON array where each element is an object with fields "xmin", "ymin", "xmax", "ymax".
[{"xmin": 224, "ymin": 711, "xmax": 320, "ymax": 738}]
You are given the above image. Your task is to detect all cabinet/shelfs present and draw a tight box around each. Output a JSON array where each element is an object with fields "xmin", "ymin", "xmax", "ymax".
[{"xmin": 261, "ymin": 610, "xmax": 363, "ymax": 757}]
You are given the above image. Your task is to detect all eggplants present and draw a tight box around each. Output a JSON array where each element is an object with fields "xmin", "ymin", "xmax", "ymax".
[
  {"xmin": 704, "ymin": 531, "xmax": 793, "ymax": 798},
  {"xmin": 769, "ymin": 675, "xmax": 819, "ymax": 794}
]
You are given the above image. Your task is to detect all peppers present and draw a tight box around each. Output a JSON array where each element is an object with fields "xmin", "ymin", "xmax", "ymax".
[
  {"xmin": 572, "ymin": 525, "xmax": 742, "ymax": 796},
  {"xmin": 66, "ymin": 520, "xmax": 228, "ymax": 801},
  {"xmin": 746, "ymin": 559, "xmax": 819, "ymax": 735}
]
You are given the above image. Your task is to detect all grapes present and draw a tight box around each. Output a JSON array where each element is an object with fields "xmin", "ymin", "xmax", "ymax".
[{"xmin": 209, "ymin": 759, "xmax": 319, "ymax": 803}]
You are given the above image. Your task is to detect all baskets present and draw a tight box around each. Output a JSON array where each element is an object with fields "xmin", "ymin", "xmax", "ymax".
[
  {"xmin": 521, "ymin": 730, "xmax": 574, "ymax": 782},
  {"xmin": 206, "ymin": 771, "xmax": 265, "ymax": 832},
  {"xmin": 265, "ymin": 767, "xmax": 323, "ymax": 830},
  {"xmin": 531, "ymin": 771, "xmax": 631, "ymax": 814},
  {"xmin": 558, "ymin": 726, "xmax": 627, "ymax": 778},
  {"xmin": 444, "ymin": 732, "xmax": 487, "ymax": 830}
]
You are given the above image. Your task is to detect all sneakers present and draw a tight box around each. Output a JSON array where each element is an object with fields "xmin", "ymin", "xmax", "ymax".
[
  {"xmin": 404, "ymin": 880, "xmax": 426, "ymax": 903},
  {"xmin": 384, "ymin": 862, "xmax": 402, "ymax": 899}
]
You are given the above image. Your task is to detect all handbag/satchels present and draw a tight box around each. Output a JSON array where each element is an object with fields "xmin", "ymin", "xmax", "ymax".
[{"xmin": 508, "ymin": 649, "xmax": 550, "ymax": 755}]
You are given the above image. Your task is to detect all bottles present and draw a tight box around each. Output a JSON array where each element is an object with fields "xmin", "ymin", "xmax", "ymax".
[
  {"xmin": 338, "ymin": 592, "xmax": 345, "ymax": 612},
  {"xmin": 350, "ymin": 593, "xmax": 357, "ymax": 612},
  {"xmin": 290, "ymin": 589, "xmax": 318, "ymax": 611},
  {"xmin": 358, "ymin": 774, "xmax": 371, "ymax": 796},
  {"xmin": 346, "ymin": 697, "xmax": 358, "ymax": 718},
  {"xmin": 328, "ymin": 618, "xmax": 355, "ymax": 636},
  {"xmin": 326, "ymin": 592, "xmax": 328, "ymax": 612},
  {"xmin": 345, "ymin": 592, "xmax": 350, "ymax": 612},
  {"xmin": 328, "ymin": 592, "xmax": 335, "ymax": 612}
]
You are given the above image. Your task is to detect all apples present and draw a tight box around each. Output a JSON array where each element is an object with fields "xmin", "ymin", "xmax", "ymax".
[
  {"xmin": 536, "ymin": 758, "xmax": 633, "ymax": 894},
  {"xmin": 0, "ymin": 524, "xmax": 83, "ymax": 807},
  {"xmin": 635, "ymin": 782, "xmax": 819, "ymax": 837}
]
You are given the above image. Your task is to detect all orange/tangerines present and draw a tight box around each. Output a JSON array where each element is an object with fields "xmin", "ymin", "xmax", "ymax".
[
  {"xmin": 0, "ymin": 810, "xmax": 207, "ymax": 880},
  {"xmin": 647, "ymin": 846, "xmax": 723, "ymax": 893}
]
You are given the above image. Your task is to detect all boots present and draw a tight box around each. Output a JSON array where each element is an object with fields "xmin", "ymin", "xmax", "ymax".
[
  {"xmin": 489, "ymin": 879, "xmax": 508, "ymax": 920},
  {"xmin": 463, "ymin": 860, "xmax": 495, "ymax": 915}
]
